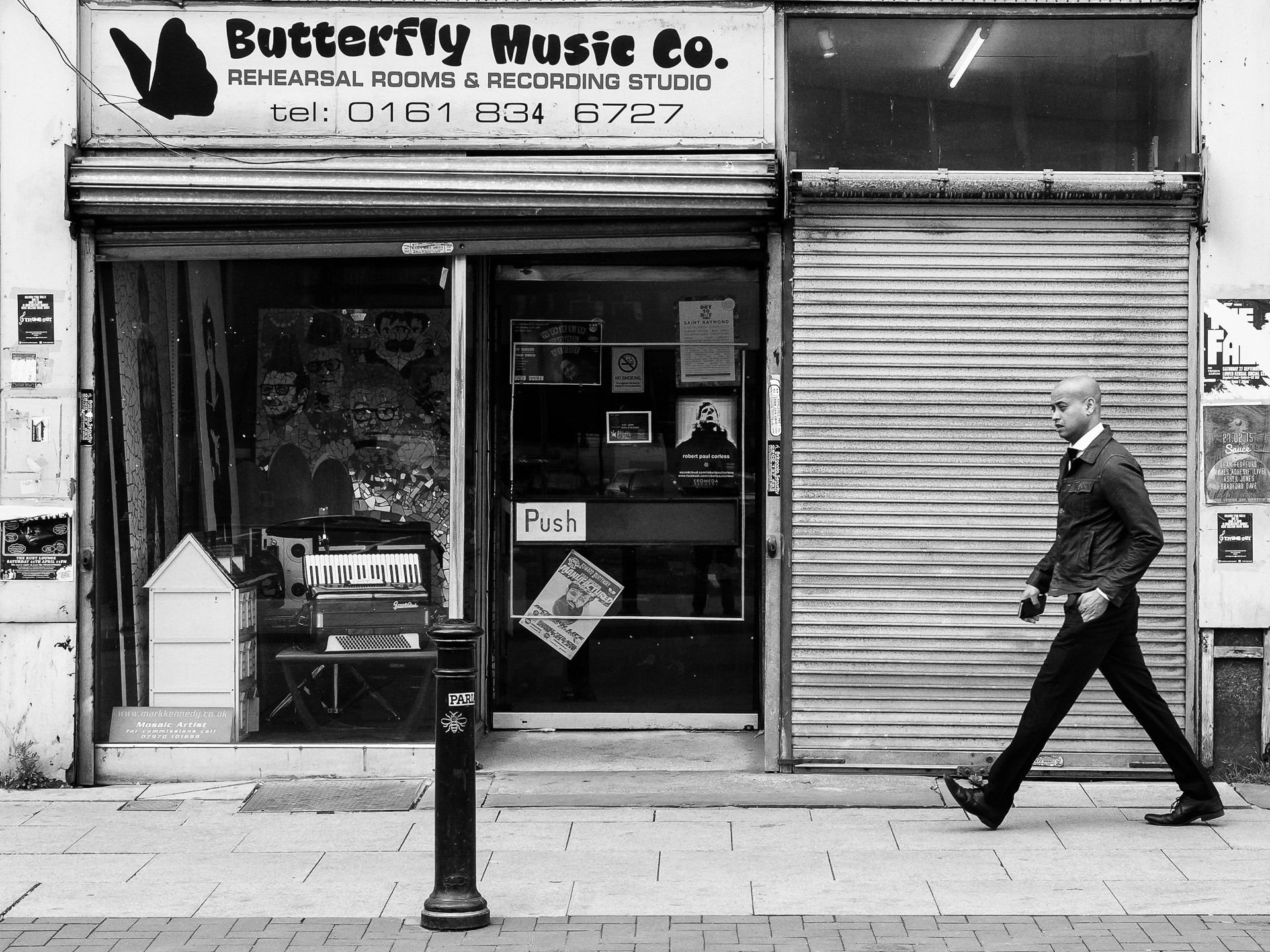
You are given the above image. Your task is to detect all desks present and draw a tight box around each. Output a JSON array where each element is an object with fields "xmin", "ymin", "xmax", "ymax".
[{"xmin": 275, "ymin": 647, "xmax": 437, "ymax": 742}]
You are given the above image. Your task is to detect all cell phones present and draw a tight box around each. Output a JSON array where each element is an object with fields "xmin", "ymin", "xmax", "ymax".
[{"xmin": 1018, "ymin": 595, "xmax": 1046, "ymax": 618}]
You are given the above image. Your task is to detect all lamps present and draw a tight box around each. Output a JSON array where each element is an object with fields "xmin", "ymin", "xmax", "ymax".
[
  {"xmin": 814, "ymin": 19, "xmax": 837, "ymax": 58},
  {"xmin": 947, "ymin": 20, "xmax": 995, "ymax": 90}
]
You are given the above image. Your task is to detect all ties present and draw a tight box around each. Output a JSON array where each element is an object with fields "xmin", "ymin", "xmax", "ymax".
[{"xmin": 1067, "ymin": 447, "xmax": 1078, "ymax": 468}]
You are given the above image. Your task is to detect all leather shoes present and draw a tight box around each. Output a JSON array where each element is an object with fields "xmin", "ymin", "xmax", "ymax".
[
  {"xmin": 943, "ymin": 774, "xmax": 1006, "ymax": 829},
  {"xmin": 1144, "ymin": 793, "xmax": 1226, "ymax": 826}
]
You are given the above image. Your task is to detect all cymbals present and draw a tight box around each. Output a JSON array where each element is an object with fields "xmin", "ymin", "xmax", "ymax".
[{"xmin": 266, "ymin": 514, "xmax": 384, "ymax": 539}]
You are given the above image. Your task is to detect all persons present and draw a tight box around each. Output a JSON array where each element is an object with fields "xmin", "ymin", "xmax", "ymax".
[
  {"xmin": 944, "ymin": 376, "xmax": 1224, "ymax": 831},
  {"xmin": 555, "ymin": 357, "xmax": 591, "ymax": 384},
  {"xmin": 676, "ymin": 402, "xmax": 736, "ymax": 466}
]
